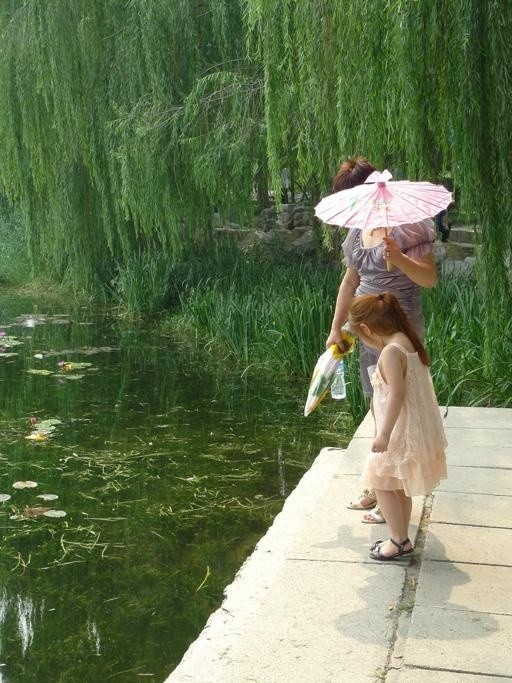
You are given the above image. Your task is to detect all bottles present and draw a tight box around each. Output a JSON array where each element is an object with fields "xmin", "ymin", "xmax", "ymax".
[{"xmin": 330, "ymin": 358, "xmax": 347, "ymax": 400}]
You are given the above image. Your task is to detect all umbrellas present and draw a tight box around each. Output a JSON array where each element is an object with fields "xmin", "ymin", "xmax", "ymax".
[
  {"xmin": 312, "ymin": 169, "xmax": 455, "ymax": 273},
  {"xmin": 303, "ymin": 320, "xmax": 359, "ymax": 418}
]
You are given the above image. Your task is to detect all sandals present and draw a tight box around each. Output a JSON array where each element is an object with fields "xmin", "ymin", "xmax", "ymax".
[
  {"xmin": 361, "ymin": 508, "xmax": 385, "ymax": 524},
  {"xmin": 346, "ymin": 489, "xmax": 376, "ymax": 510},
  {"xmin": 370, "ymin": 538, "xmax": 414, "ymax": 561}
]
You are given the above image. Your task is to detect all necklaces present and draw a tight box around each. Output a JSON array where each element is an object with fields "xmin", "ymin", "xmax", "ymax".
[{"xmin": 368, "ymin": 227, "xmax": 382, "ymax": 236}]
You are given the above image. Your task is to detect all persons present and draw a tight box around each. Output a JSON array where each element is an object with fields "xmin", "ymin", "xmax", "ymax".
[
  {"xmin": 346, "ymin": 291, "xmax": 449, "ymax": 562},
  {"xmin": 325, "ymin": 157, "xmax": 437, "ymax": 525}
]
